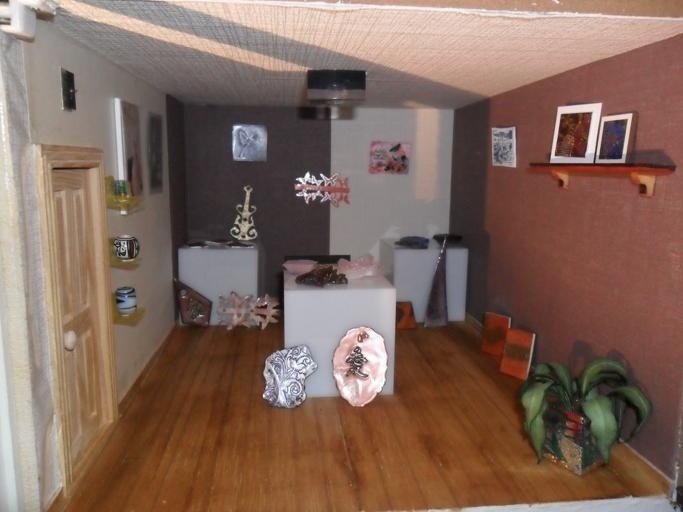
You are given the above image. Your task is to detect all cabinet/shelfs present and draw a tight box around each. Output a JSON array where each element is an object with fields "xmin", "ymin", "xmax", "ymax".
[{"xmin": 108, "ymin": 199, "xmax": 148, "ymax": 326}]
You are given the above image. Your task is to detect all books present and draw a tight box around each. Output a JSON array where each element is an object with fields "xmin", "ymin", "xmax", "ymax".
[
  {"xmin": 481, "ymin": 311, "xmax": 511, "ymax": 359},
  {"xmin": 395, "ymin": 300, "xmax": 418, "ymax": 330},
  {"xmin": 500, "ymin": 328, "xmax": 536, "ymax": 382}
]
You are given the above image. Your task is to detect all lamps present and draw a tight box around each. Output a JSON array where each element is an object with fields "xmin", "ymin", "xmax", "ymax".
[{"xmin": 305, "ymin": 69, "xmax": 368, "ymax": 103}]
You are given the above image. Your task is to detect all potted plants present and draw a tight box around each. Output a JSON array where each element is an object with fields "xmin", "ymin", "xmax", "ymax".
[{"xmin": 516, "ymin": 353, "xmax": 654, "ymax": 478}]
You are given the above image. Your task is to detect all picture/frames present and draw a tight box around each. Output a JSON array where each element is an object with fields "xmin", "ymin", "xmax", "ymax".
[{"xmin": 549, "ymin": 103, "xmax": 637, "ymax": 163}]
